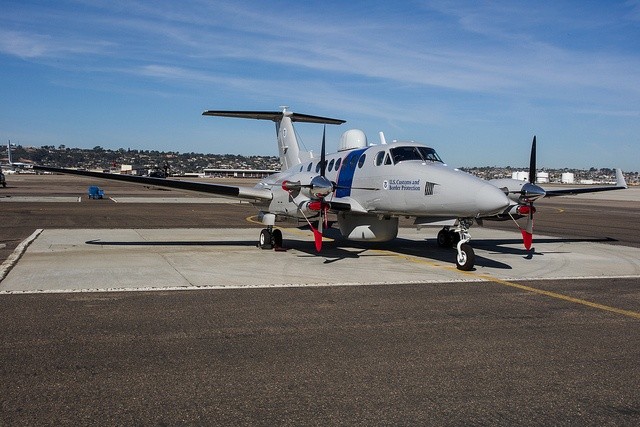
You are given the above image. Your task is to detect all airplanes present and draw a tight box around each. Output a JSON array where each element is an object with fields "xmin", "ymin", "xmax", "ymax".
[{"xmin": 6, "ymin": 104, "xmax": 628, "ymax": 272}]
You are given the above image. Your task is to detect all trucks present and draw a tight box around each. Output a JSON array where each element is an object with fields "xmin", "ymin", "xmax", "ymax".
[{"xmin": 88, "ymin": 186, "xmax": 104, "ymax": 200}]
